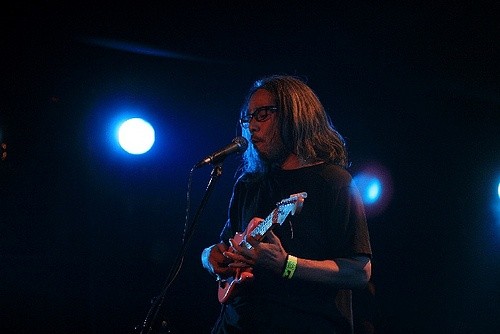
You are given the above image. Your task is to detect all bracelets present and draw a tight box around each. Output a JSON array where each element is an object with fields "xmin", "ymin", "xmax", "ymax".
[{"xmin": 282, "ymin": 254, "xmax": 298, "ymax": 279}]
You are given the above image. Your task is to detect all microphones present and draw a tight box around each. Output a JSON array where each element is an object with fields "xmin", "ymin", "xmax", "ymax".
[{"xmin": 195, "ymin": 137, "xmax": 248, "ymax": 168}]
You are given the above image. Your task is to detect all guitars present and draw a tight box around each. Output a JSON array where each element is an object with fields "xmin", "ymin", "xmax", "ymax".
[{"xmin": 218, "ymin": 192, "xmax": 308, "ymax": 303}]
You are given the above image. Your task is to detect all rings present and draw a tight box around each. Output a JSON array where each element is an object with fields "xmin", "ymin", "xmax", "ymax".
[{"xmin": 240, "ymin": 250, "xmax": 252, "ymax": 259}]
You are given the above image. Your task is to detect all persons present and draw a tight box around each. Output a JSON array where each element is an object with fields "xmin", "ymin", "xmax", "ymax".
[{"xmin": 201, "ymin": 76, "xmax": 372, "ymax": 334}]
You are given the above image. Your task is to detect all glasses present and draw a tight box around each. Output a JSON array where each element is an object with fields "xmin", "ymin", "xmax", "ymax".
[{"xmin": 239, "ymin": 106, "xmax": 280, "ymax": 129}]
